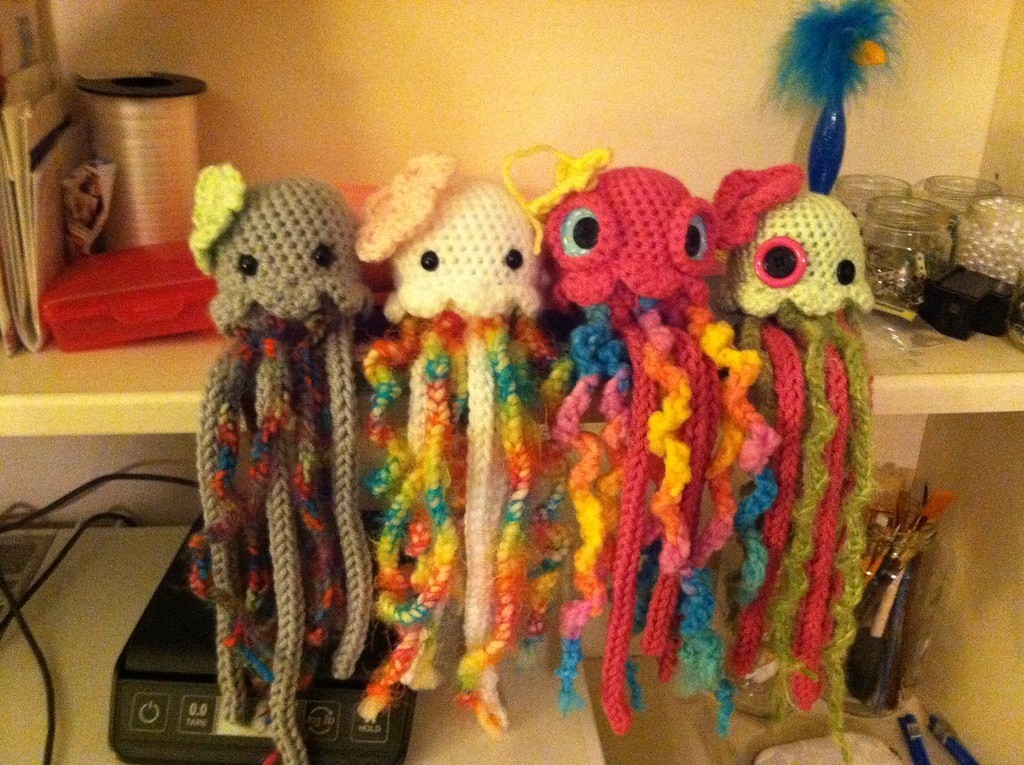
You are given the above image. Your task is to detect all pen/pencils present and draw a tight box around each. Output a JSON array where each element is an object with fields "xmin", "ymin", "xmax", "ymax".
[
  {"xmin": 929, "ymin": 714, "xmax": 979, "ymax": 765},
  {"xmin": 897, "ymin": 712, "xmax": 932, "ymax": 765},
  {"xmin": 854, "ymin": 479, "xmax": 956, "ymax": 710}
]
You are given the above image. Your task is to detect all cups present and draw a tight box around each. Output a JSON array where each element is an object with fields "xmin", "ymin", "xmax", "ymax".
[{"xmin": 844, "ymin": 542, "xmax": 919, "ymax": 717}]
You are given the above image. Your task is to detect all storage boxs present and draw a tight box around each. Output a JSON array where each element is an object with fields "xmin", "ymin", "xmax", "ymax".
[{"xmin": 40, "ymin": 242, "xmax": 218, "ymax": 351}]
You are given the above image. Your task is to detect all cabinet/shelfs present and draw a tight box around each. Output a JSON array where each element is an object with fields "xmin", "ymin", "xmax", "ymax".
[{"xmin": 1, "ymin": 2, "xmax": 1024, "ymax": 764}]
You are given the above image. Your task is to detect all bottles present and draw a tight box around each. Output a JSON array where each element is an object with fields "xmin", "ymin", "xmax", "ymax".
[
  {"xmin": 918, "ymin": 176, "xmax": 1001, "ymax": 231},
  {"xmin": 864, "ymin": 197, "xmax": 949, "ymax": 311},
  {"xmin": 952, "ymin": 195, "xmax": 1024, "ymax": 286},
  {"xmin": 835, "ymin": 176, "xmax": 911, "ymax": 224}
]
